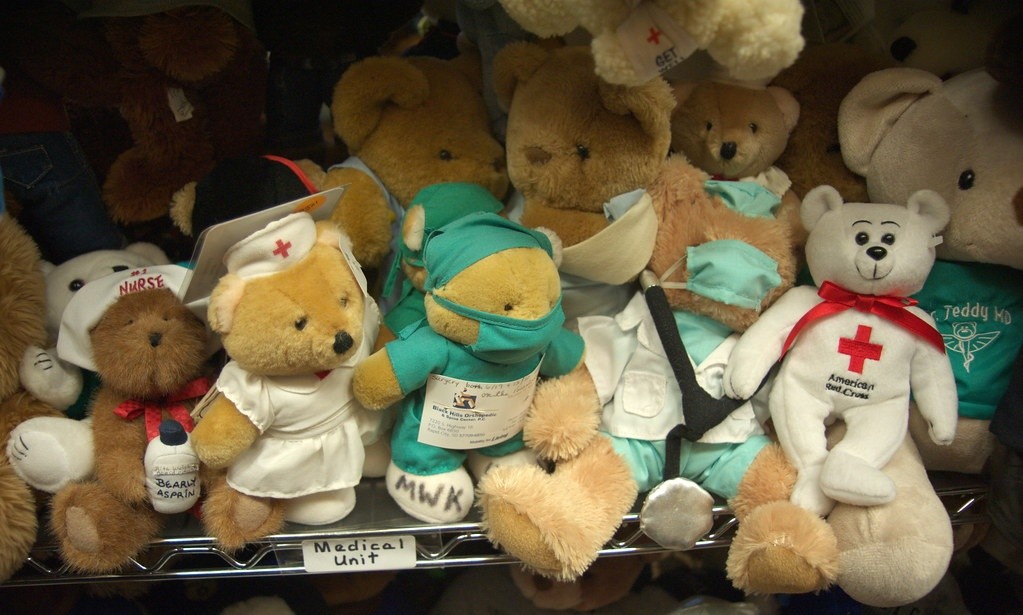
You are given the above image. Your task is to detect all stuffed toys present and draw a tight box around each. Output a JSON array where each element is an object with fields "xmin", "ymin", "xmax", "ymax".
[{"xmin": 0, "ymin": 0, "xmax": 1023, "ymax": 615}]
